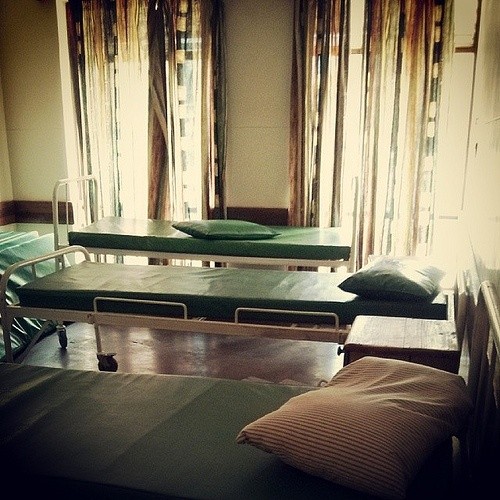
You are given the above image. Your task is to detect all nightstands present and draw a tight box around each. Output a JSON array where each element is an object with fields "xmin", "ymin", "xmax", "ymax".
[{"xmin": 338, "ymin": 315, "xmax": 460, "ymax": 375}]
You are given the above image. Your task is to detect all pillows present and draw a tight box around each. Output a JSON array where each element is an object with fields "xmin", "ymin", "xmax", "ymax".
[
  {"xmin": 337, "ymin": 255, "xmax": 450, "ymax": 300},
  {"xmin": 235, "ymin": 355, "xmax": 471, "ymax": 500},
  {"xmin": 172, "ymin": 219, "xmax": 282, "ymax": 240}
]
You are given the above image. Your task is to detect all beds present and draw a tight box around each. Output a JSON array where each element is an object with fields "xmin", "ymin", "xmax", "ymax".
[
  {"xmin": 0, "ymin": 245, "xmax": 468, "ymax": 371},
  {"xmin": 52, "ymin": 174, "xmax": 360, "ymax": 272},
  {"xmin": 0, "ymin": 281, "xmax": 500, "ymax": 500}
]
